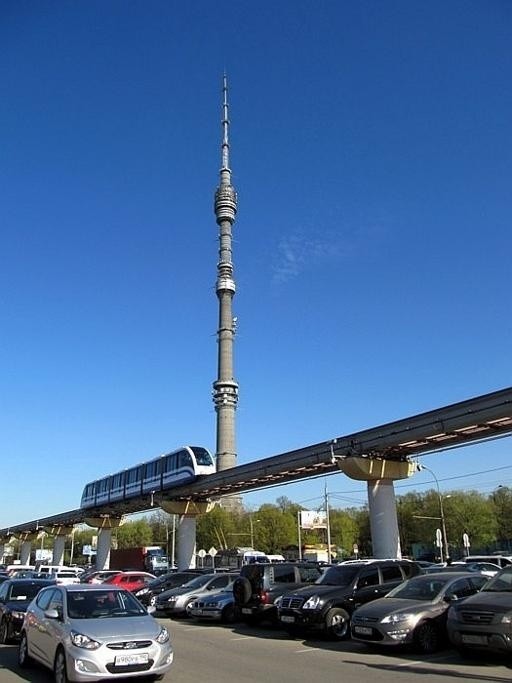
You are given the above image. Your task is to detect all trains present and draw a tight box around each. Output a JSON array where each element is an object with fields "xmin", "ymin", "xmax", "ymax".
[{"xmin": 78, "ymin": 444, "xmax": 216, "ymax": 509}]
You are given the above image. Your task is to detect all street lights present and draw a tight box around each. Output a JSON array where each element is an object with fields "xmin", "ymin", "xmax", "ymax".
[
  {"xmin": 416, "ymin": 464, "xmax": 452, "ymax": 560},
  {"xmin": 249, "ymin": 518, "xmax": 261, "ymax": 549}
]
